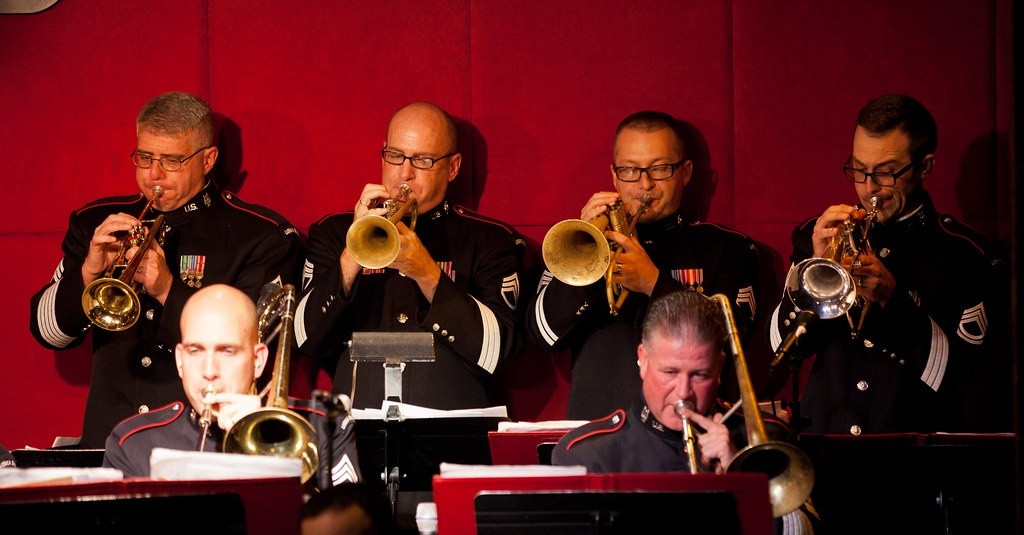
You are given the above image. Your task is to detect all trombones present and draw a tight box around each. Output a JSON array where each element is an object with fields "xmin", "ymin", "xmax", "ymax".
[
  {"xmin": 191, "ymin": 282, "xmax": 320, "ymax": 482},
  {"xmin": 674, "ymin": 290, "xmax": 817, "ymax": 517}
]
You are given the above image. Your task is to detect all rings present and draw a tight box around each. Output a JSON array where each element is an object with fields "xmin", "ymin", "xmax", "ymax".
[
  {"xmin": 360, "ymin": 200, "xmax": 367, "ymax": 206},
  {"xmin": 232, "ymin": 415, "xmax": 240, "ymax": 423},
  {"xmin": 859, "ymin": 277, "xmax": 863, "ymax": 287},
  {"xmin": 137, "ymin": 266, "xmax": 144, "ymax": 273},
  {"xmin": 616, "ymin": 263, "xmax": 624, "ymax": 273}
]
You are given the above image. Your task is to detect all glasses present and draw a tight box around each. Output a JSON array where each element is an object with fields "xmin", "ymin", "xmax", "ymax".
[
  {"xmin": 380, "ymin": 146, "xmax": 460, "ymax": 170},
  {"xmin": 129, "ymin": 146, "xmax": 208, "ymax": 172},
  {"xmin": 611, "ymin": 159, "xmax": 686, "ymax": 182},
  {"xmin": 842, "ymin": 153, "xmax": 916, "ymax": 186}
]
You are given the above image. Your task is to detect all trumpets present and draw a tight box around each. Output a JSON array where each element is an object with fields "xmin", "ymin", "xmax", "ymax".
[
  {"xmin": 80, "ymin": 184, "xmax": 166, "ymax": 334},
  {"xmin": 344, "ymin": 184, "xmax": 416, "ymax": 269},
  {"xmin": 785, "ymin": 196, "xmax": 882, "ymax": 322},
  {"xmin": 542, "ymin": 194, "xmax": 649, "ymax": 317}
]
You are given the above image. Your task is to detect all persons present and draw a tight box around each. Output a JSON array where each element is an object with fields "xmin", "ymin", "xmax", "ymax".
[
  {"xmin": 522, "ymin": 110, "xmax": 778, "ymax": 425},
  {"xmin": 547, "ymin": 289, "xmax": 795, "ymax": 473},
  {"xmin": 290, "ymin": 98, "xmax": 533, "ymax": 497},
  {"xmin": 758, "ymin": 92, "xmax": 1010, "ymax": 535},
  {"xmin": 98, "ymin": 281, "xmax": 388, "ymax": 535},
  {"xmin": 30, "ymin": 86, "xmax": 307, "ymax": 452}
]
